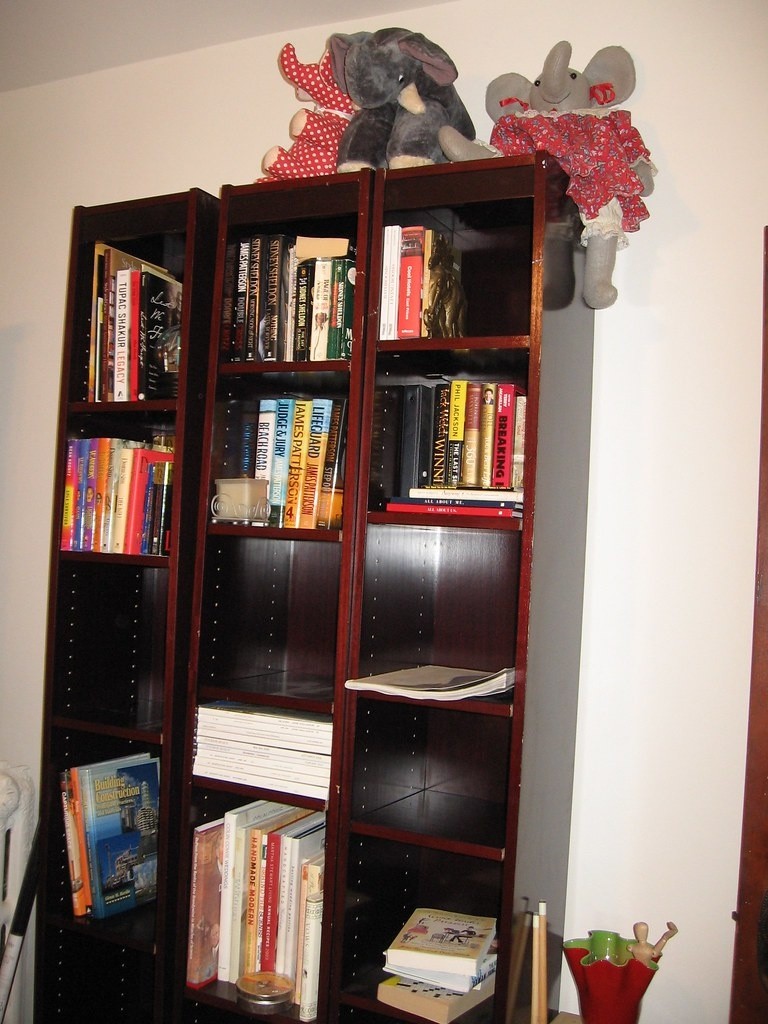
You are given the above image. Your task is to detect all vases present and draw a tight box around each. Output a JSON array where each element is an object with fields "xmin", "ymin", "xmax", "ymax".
[{"xmin": 563, "ymin": 930, "xmax": 663, "ymax": 1024}]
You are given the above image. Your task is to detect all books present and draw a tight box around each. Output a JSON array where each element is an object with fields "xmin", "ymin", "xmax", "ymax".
[
  {"xmin": 48, "ymin": 758, "xmax": 158, "ymax": 921},
  {"xmin": 188, "ymin": 692, "xmax": 330, "ymax": 800},
  {"xmin": 60, "ymin": 434, "xmax": 171, "ymax": 557},
  {"xmin": 184, "ymin": 800, "xmax": 325, "ymax": 1023},
  {"xmin": 225, "ymin": 232, "xmax": 355, "ymax": 361},
  {"xmin": 376, "ymin": 906, "xmax": 497, "ymax": 1023},
  {"xmin": 382, "ymin": 228, "xmax": 466, "ymax": 338},
  {"xmin": 383, "ymin": 369, "xmax": 530, "ymax": 521},
  {"xmin": 238, "ymin": 393, "xmax": 345, "ymax": 529},
  {"xmin": 337, "ymin": 655, "xmax": 526, "ymax": 702},
  {"xmin": 89, "ymin": 244, "xmax": 182, "ymax": 403}
]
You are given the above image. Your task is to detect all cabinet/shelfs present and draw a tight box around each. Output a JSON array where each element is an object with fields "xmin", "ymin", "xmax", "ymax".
[{"xmin": 32, "ymin": 163, "xmax": 596, "ymax": 1024}]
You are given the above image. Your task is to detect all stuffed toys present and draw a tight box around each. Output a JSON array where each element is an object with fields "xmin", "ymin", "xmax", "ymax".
[
  {"xmin": 256, "ymin": 29, "xmax": 475, "ymax": 176},
  {"xmin": 442, "ymin": 40, "xmax": 655, "ymax": 312}
]
